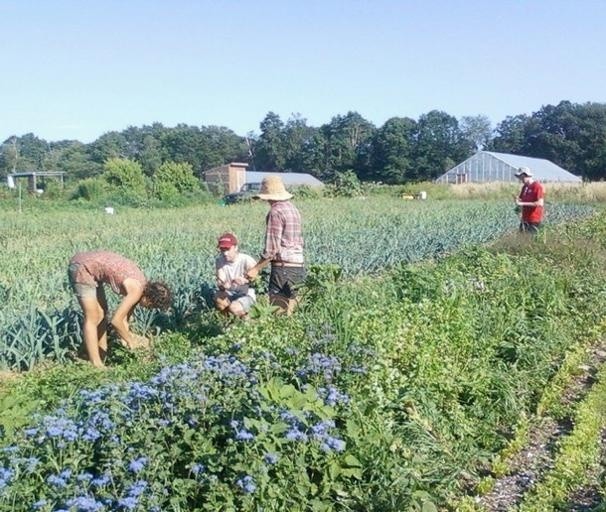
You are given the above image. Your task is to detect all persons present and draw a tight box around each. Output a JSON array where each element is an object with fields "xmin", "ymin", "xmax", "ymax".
[
  {"xmin": 512, "ymin": 166, "xmax": 546, "ymax": 234},
  {"xmin": 243, "ymin": 175, "xmax": 306, "ymax": 318},
  {"xmin": 66, "ymin": 249, "xmax": 173, "ymax": 374},
  {"xmin": 212, "ymin": 233, "xmax": 261, "ymax": 323}
]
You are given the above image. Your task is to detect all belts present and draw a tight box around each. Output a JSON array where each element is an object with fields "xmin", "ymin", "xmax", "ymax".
[{"xmin": 271, "ymin": 262, "xmax": 304, "ymax": 268}]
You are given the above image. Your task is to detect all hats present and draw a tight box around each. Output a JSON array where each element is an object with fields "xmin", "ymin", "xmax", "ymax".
[
  {"xmin": 250, "ymin": 176, "xmax": 294, "ymax": 202},
  {"xmin": 216, "ymin": 233, "xmax": 238, "ymax": 249},
  {"xmin": 514, "ymin": 167, "xmax": 532, "ymax": 178}
]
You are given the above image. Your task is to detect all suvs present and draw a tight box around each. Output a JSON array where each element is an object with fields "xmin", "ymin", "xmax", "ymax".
[{"xmin": 222, "ymin": 180, "xmax": 262, "ymax": 206}]
